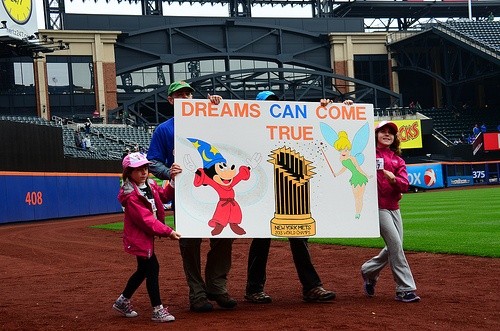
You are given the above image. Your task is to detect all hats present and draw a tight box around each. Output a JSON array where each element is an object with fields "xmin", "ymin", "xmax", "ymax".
[
  {"xmin": 375, "ymin": 121, "xmax": 398, "ymax": 135},
  {"xmin": 255, "ymin": 91, "xmax": 279, "ymax": 101},
  {"xmin": 168, "ymin": 81, "xmax": 196, "ymax": 96},
  {"xmin": 122, "ymin": 152, "xmax": 153, "ymax": 168}
]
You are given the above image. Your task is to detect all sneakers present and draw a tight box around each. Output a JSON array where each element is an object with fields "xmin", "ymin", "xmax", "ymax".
[
  {"xmin": 395, "ymin": 291, "xmax": 421, "ymax": 303},
  {"xmin": 360, "ymin": 269, "xmax": 375, "ymax": 297},
  {"xmin": 150, "ymin": 304, "xmax": 176, "ymax": 321},
  {"xmin": 243, "ymin": 292, "xmax": 271, "ymax": 303},
  {"xmin": 303, "ymin": 287, "xmax": 335, "ymax": 301},
  {"xmin": 113, "ymin": 294, "xmax": 138, "ymax": 318}
]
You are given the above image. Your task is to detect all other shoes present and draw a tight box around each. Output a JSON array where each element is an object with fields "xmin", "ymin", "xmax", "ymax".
[
  {"xmin": 214, "ymin": 294, "xmax": 238, "ymax": 308},
  {"xmin": 191, "ymin": 299, "xmax": 213, "ymax": 313}
]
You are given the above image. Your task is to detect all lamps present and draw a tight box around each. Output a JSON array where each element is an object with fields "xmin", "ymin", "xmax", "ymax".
[
  {"xmin": 23, "ymin": 32, "xmax": 70, "ymax": 51},
  {"xmin": 0, "ymin": 21, "xmax": 8, "ymax": 29}
]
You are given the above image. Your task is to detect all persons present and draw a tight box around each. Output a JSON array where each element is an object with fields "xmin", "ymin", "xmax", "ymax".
[
  {"xmin": 245, "ymin": 90, "xmax": 337, "ymax": 304},
  {"xmin": 113, "ymin": 152, "xmax": 181, "ymax": 323},
  {"xmin": 147, "ymin": 81, "xmax": 240, "ymax": 311},
  {"xmin": 74, "ymin": 117, "xmax": 92, "ymax": 150},
  {"xmin": 342, "ymin": 100, "xmax": 421, "ymax": 301},
  {"xmin": 408, "ymin": 101, "xmax": 420, "ymax": 114}
]
